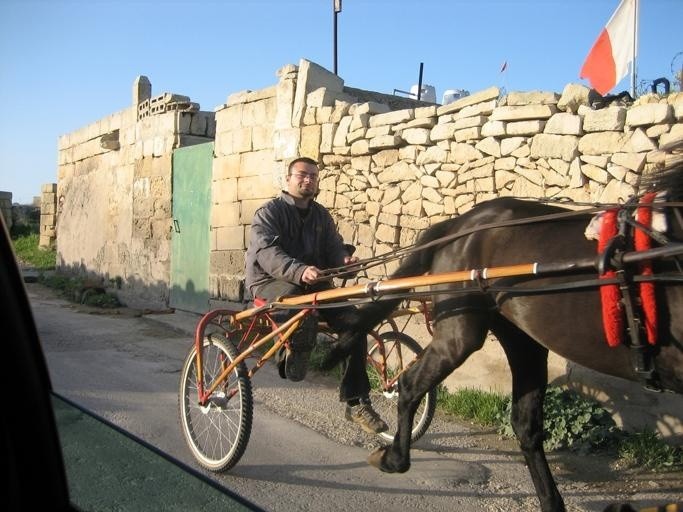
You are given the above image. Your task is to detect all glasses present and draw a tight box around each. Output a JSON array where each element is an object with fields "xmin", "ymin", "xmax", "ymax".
[{"xmin": 288, "ymin": 172, "xmax": 318, "ymax": 179}]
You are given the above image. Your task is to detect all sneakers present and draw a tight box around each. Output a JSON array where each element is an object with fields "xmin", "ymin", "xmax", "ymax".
[
  {"xmin": 285, "ymin": 314, "xmax": 318, "ymax": 382},
  {"xmin": 345, "ymin": 396, "xmax": 389, "ymax": 434}
]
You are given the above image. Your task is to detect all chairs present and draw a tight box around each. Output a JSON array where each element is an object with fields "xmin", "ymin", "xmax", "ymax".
[{"xmin": 236, "ymin": 244, "xmax": 355, "ymax": 328}]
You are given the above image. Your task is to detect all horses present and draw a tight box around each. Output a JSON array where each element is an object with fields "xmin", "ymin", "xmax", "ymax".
[{"xmin": 318, "ymin": 159, "xmax": 683, "ymax": 512}]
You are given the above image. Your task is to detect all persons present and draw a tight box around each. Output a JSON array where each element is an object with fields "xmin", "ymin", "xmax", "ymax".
[{"xmin": 244, "ymin": 157, "xmax": 389, "ymax": 434}]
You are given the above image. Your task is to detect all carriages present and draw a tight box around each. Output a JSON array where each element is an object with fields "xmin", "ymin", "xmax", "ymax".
[{"xmin": 172, "ymin": 136, "xmax": 682, "ymax": 512}]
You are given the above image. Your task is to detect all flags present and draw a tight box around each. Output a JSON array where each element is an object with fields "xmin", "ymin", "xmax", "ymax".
[{"xmin": 578, "ymin": 0, "xmax": 639, "ymax": 98}]
